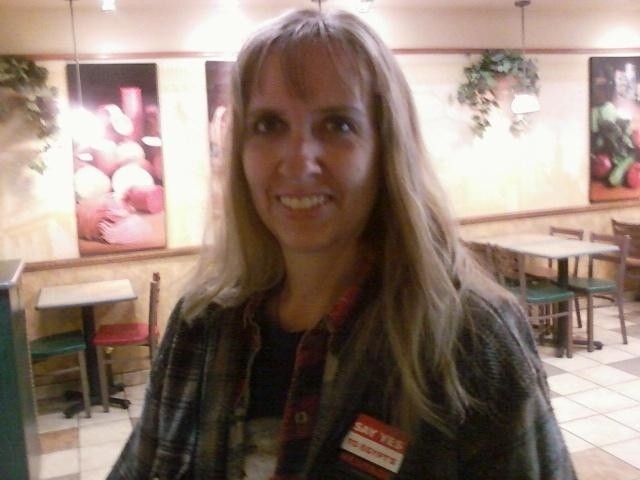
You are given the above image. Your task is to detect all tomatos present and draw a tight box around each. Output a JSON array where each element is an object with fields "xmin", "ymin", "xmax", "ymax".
[
  {"xmin": 630, "ymin": 128, "xmax": 640, "ymax": 149},
  {"xmin": 627, "ymin": 163, "xmax": 640, "ymax": 189}
]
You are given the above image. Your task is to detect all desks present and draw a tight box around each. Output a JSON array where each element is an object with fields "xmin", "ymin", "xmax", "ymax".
[{"xmin": 36, "ymin": 276, "xmax": 140, "ymax": 421}]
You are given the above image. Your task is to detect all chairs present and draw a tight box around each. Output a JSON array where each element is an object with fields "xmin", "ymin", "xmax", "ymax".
[
  {"xmin": 91, "ymin": 270, "xmax": 164, "ymax": 412},
  {"xmin": 457, "ymin": 217, "xmax": 640, "ymax": 359}
]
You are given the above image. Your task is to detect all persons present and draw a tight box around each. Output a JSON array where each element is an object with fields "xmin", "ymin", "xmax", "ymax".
[{"xmin": 99, "ymin": 6, "xmax": 579, "ymax": 480}]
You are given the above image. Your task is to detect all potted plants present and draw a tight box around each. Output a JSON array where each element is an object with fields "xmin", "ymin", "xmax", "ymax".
[
  {"xmin": 0, "ymin": 53, "xmax": 61, "ymax": 174},
  {"xmin": 456, "ymin": 45, "xmax": 540, "ymax": 139}
]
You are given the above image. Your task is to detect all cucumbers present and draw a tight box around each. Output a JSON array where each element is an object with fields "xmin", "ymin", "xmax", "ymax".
[{"xmin": 590, "ymin": 118, "xmax": 637, "ymax": 187}]
